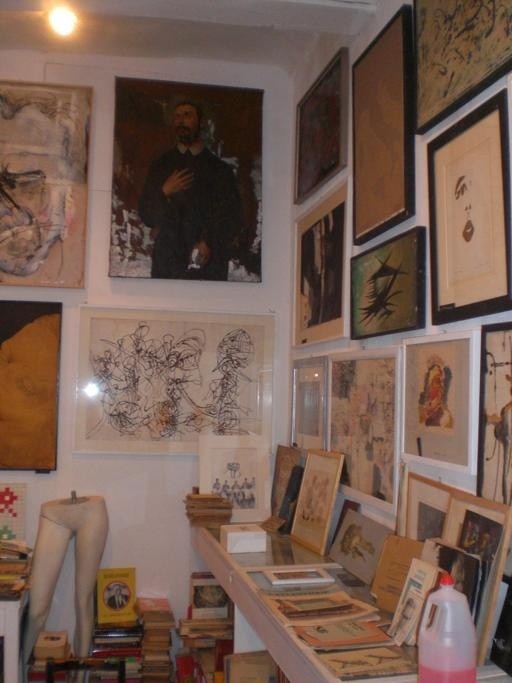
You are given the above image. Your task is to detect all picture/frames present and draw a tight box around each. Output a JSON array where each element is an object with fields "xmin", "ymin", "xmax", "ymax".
[
  {"xmin": 73, "ymin": 303, "xmax": 277, "ymax": 458},
  {"xmin": 0, "ymin": 81, "xmax": 92, "ymax": 289}
]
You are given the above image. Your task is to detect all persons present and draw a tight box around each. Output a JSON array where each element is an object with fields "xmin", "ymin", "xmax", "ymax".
[
  {"xmin": 138, "ymin": 99, "xmax": 242, "ymax": 280},
  {"xmin": 211, "ymin": 477, "xmax": 256, "ymax": 506},
  {"xmin": 19, "ymin": 489, "xmax": 110, "ymax": 683}
]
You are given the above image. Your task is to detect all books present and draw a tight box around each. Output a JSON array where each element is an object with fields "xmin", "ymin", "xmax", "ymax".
[
  {"xmin": 35, "ymin": 630, "xmax": 79, "ymax": 671},
  {"xmin": 182, "ymin": 492, "xmax": 232, "ymax": 528},
  {"xmin": 1, "ymin": 537, "xmax": 36, "ymax": 601},
  {"xmin": 262, "ymin": 533, "xmax": 481, "ymax": 681},
  {"xmin": 88, "ymin": 565, "xmax": 276, "ymax": 683}
]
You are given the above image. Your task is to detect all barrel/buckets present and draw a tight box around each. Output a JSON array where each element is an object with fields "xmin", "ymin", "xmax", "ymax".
[{"xmin": 417, "ymin": 575, "xmax": 477, "ymax": 682}]
